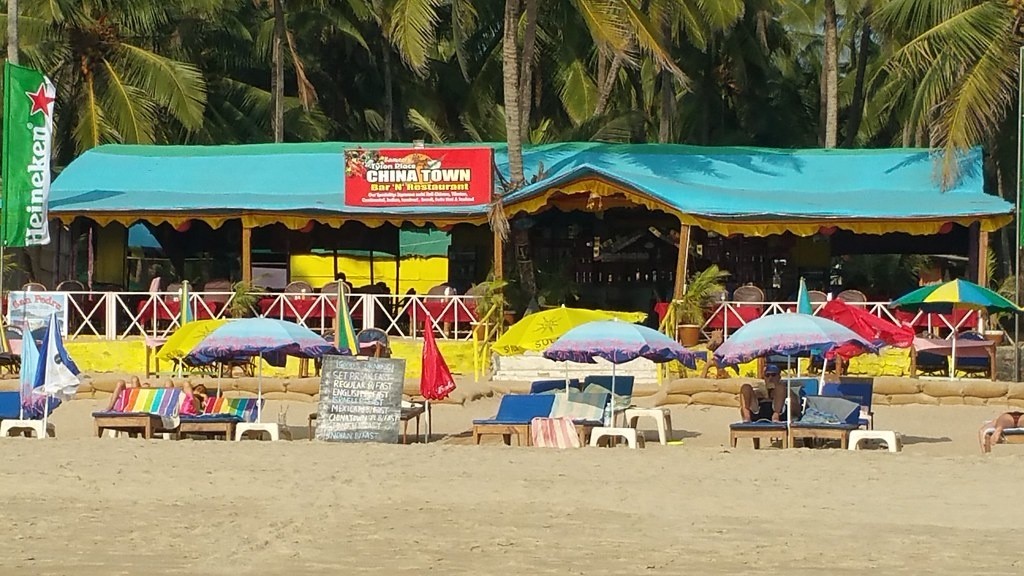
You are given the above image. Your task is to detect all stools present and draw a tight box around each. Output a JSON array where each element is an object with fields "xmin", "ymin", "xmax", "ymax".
[
  {"xmin": 1, "ymin": 419, "xmax": 56, "ymax": 441},
  {"xmin": 234, "ymin": 421, "xmax": 293, "ymax": 441},
  {"xmin": 620, "ymin": 405, "xmax": 673, "ymax": 445},
  {"xmin": 588, "ymin": 426, "xmax": 646, "ymax": 450},
  {"xmin": 847, "ymin": 429, "xmax": 902, "ymax": 454}
]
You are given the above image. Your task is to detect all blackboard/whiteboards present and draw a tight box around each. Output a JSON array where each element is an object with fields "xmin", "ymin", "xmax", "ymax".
[{"xmin": 313, "ymin": 353, "xmax": 407, "ymax": 445}]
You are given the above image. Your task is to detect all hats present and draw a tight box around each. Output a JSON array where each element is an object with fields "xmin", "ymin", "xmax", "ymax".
[{"xmin": 764, "ymin": 364, "xmax": 781, "ymax": 372}]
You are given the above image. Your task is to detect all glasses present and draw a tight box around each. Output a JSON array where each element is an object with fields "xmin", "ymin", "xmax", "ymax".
[{"xmin": 764, "ymin": 373, "xmax": 777, "ymax": 378}]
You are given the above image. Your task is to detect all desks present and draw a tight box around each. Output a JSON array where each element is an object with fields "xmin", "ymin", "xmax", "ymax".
[
  {"xmin": 308, "ymin": 400, "xmax": 432, "ymax": 445},
  {"xmin": 298, "ymin": 340, "xmax": 391, "ymax": 378},
  {"xmin": 894, "ymin": 303, "xmax": 979, "ymax": 338},
  {"xmin": 144, "ymin": 335, "xmax": 251, "ymax": 380},
  {"xmin": 909, "ymin": 335, "xmax": 998, "ymax": 382},
  {"xmin": 654, "ymin": 302, "xmax": 762, "ymax": 341}
]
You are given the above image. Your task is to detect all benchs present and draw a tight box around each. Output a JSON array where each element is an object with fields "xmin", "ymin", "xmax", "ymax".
[
  {"xmin": 0, "ymin": 390, "xmax": 63, "ymax": 440},
  {"xmin": 530, "ymin": 376, "xmax": 579, "ymax": 396},
  {"xmin": 982, "ymin": 420, "xmax": 1024, "ymax": 445},
  {"xmin": 808, "ymin": 353, "xmax": 849, "ymax": 377},
  {"xmin": 176, "ymin": 396, "xmax": 265, "ymax": 442},
  {"xmin": 472, "ymin": 393, "xmax": 556, "ymax": 446},
  {"xmin": 583, "ymin": 375, "xmax": 637, "ymax": 444},
  {"xmin": 789, "ymin": 383, "xmax": 874, "ymax": 450},
  {"xmin": 756, "ymin": 354, "xmax": 798, "ymax": 379},
  {"xmin": 91, "ymin": 386, "xmax": 186, "ymax": 439},
  {"xmin": 730, "ymin": 379, "xmax": 819, "ymax": 450},
  {"xmin": 531, "ymin": 391, "xmax": 612, "ymax": 448}
]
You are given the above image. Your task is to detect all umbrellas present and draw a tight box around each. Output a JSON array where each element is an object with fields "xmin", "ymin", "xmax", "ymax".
[
  {"xmin": 189, "ymin": 313, "xmax": 333, "ymax": 442},
  {"xmin": 710, "ymin": 314, "xmax": 880, "ymax": 450},
  {"xmin": 420, "ymin": 312, "xmax": 456, "ymax": 448},
  {"xmin": 333, "ymin": 277, "xmax": 360, "ymax": 358},
  {"xmin": 792, "ymin": 278, "xmax": 823, "ymax": 357},
  {"xmin": 542, "ymin": 317, "xmax": 698, "ymax": 447},
  {"xmin": 491, "ymin": 301, "xmax": 649, "ymax": 398},
  {"xmin": 157, "ymin": 314, "xmax": 244, "ymax": 398},
  {"xmin": 886, "ymin": 277, "xmax": 1024, "ymax": 380},
  {"xmin": 21, "ymin": 317, "xmax": 49, "ymax": 417},
  {"xmin": 180, "ymin": 279, "xmax": 194, "ymax": 326},
  {"xmin": 32, "ymin": 309, "xmax": 84, "ymax": 403},
  {"xmin": 809, "ymin": 296, "xmax": 912, "ymax": 391}
]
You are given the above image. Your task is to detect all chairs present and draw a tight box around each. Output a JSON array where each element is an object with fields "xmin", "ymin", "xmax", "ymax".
[
  {"xmin": 952, "ymin": 330, "xmax": 992, "ymax": 379},
  {"xmin": 313, "ymin": 329, "xmax": 336, "ymax": 378},
  {"xmin": 909, "ymin": 330, "xmax": 950, "ymax": 377},
  {"xmin": 357, "ymin": 328, "xmax": 389, "ymax": 348},
  {"xmin": 703, "ymin": 285, "xmax": 867, "ymax": 312}
]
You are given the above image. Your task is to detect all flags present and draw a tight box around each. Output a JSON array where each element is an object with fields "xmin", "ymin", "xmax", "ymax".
[{"xmin": 5, "ymin": 59, "xmax": 53, "ymax": 248}]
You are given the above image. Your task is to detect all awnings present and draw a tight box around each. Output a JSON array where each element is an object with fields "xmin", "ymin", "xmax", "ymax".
[{"xmin": 44, "ymin": 130, "xmax": 1020, "ymax": 338}]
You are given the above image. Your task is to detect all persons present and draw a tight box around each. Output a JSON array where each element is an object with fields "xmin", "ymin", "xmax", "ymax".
[
  {"xmin": 979, "ymin": 405, "xmax": 1024, "ymax": 454},
  {"xmin": 736, "ymin": 361, "xmax": 802, "ymax": 425},
  {"xmin": 103, "ymin": 374, "xmax": 149, "ymax": 412},
  {"xmin": 162, "ymin": 377, "xmax": 207, "ymax": 418}
]
